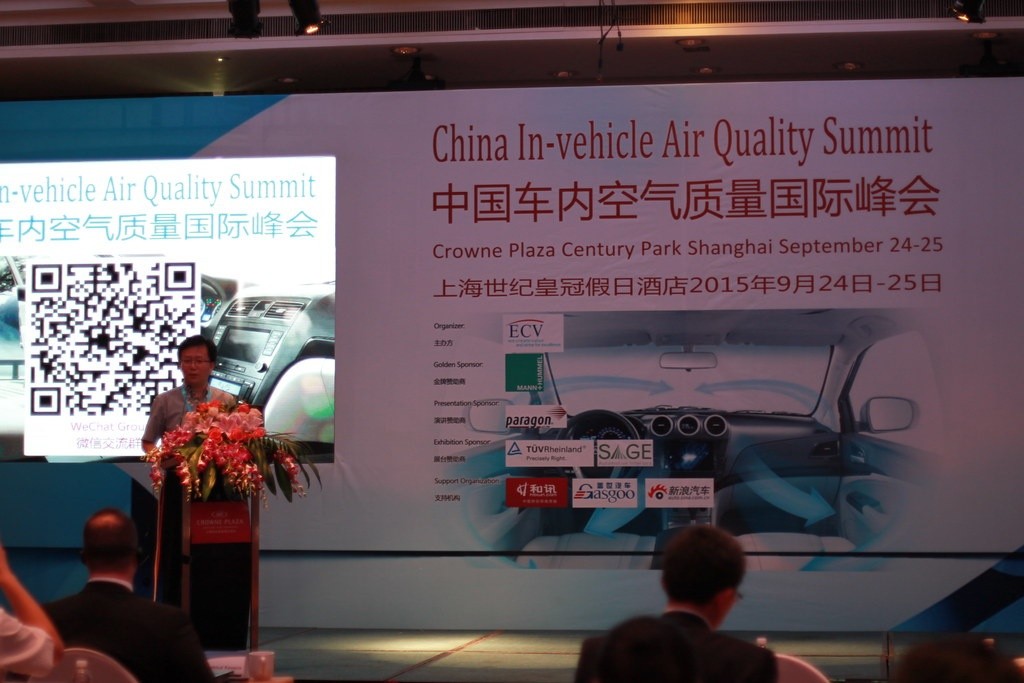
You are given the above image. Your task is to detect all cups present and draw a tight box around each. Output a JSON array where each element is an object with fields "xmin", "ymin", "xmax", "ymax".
[{"xmin": 248, "ymin": 651, "xmax": 275, "ymax": 681}]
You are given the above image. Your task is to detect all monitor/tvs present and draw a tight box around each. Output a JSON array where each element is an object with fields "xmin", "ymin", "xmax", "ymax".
[
  {"xmin": 218, "ymin": 327, "xmax": 270, "ymax": 364},
  {"xmin": 662, "ymin": 439, "xmax": 715, "ymax": 470}
]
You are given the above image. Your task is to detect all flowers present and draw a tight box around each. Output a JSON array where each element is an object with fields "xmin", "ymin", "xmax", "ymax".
[{"xmin": 147, "ymin": 397, "xmax": 323, "ymax": 510}]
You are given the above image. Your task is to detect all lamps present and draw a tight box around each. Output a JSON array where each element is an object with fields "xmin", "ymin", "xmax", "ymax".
[
  {"xmin": 949, "ymin": 0, "xmax": 987, "ymax": 23},
  {"xmin": 288, "ymin": 0, "xmax": 332, "ymax": 37},
  {"xmin": 225, "ymin": 0, "xmax": 264, "ymax": 38}
]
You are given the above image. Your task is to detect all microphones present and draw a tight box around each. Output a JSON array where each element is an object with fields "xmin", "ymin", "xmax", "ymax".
[{"xmin": 180, "ymin": 384, "xmax": 192, "ymax": 426}]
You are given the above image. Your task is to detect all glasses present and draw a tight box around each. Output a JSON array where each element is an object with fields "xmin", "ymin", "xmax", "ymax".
[{"xmin": 181, "ymin": 358, "xmax": 212, "ymax": 364}]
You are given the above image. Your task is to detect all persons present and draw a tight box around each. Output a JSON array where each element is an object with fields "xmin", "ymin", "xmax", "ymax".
[
  {"xmin": 578, "ymin": 525, "xmax": 1021, "ymax": 683},
  {"xmin": 55, "ymin": 509, "xmax": 216, "ymax": 683},
  {"xmin": 0, "ymin": 546, "xmax": 62, "ymax": 676},
  {"xmin": 141, "ymin": 335, "xmax": 235, "ymax": 455}
]
